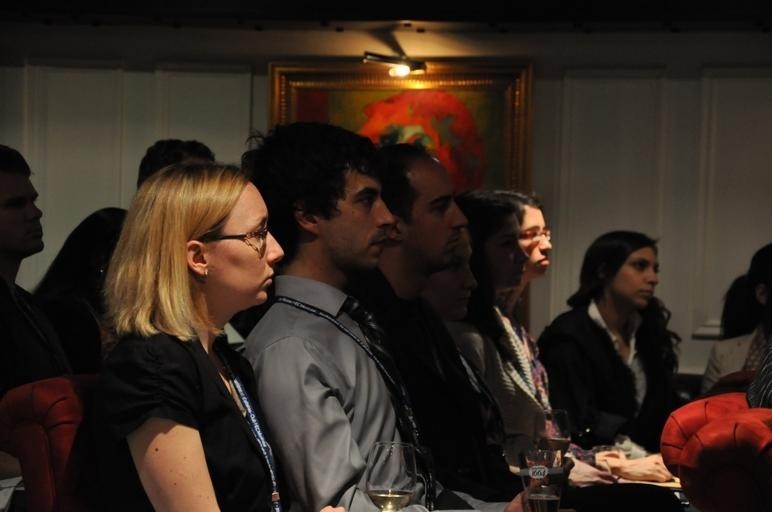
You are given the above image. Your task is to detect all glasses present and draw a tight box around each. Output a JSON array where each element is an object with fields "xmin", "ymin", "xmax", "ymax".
[
  {"xmin": 518, "ymin": 229, "xmax": 552, "ymax": 242},
  {"xmin": 198, "ymin": 225, "xmax": 274, "ymax": 244}
]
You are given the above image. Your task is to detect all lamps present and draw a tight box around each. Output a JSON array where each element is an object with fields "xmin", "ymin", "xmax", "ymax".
[{"xmin": 362, "ymin": 51, "xmax": 428, "ymax": 79}]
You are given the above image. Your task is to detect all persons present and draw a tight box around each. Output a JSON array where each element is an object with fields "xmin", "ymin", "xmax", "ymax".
[
  {"xmin": 58, "ymin": 117, "xmax": 683, "ymax": 512},
  {"xmin": 31, "ymin": 138, "xmax": 216, "ymax": 382},
  {"xmin": 0, "ymin": 143, "xmax": 79, "ymax": 396},
  {"xmin": 699, "ymin": 243, "xmax": 772, "ymax": 399}
]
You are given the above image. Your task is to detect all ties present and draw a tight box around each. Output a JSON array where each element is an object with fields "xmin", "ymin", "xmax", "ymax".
[{"xmin": 344, "ymin": 296, "xmax": 436, "ymax": 512}]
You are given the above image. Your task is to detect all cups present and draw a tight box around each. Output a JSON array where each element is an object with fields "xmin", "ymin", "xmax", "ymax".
[
  {"xmin": 518, "ymin": 449, "xmax": 562, "ymax": 511},
  {"xmin": 364, "ymin": 439, "xmax": 418, "ymax": 512},
  {"xmin": 534, "ymin": 408, "xmax": 571, "ymax": 456}
]
